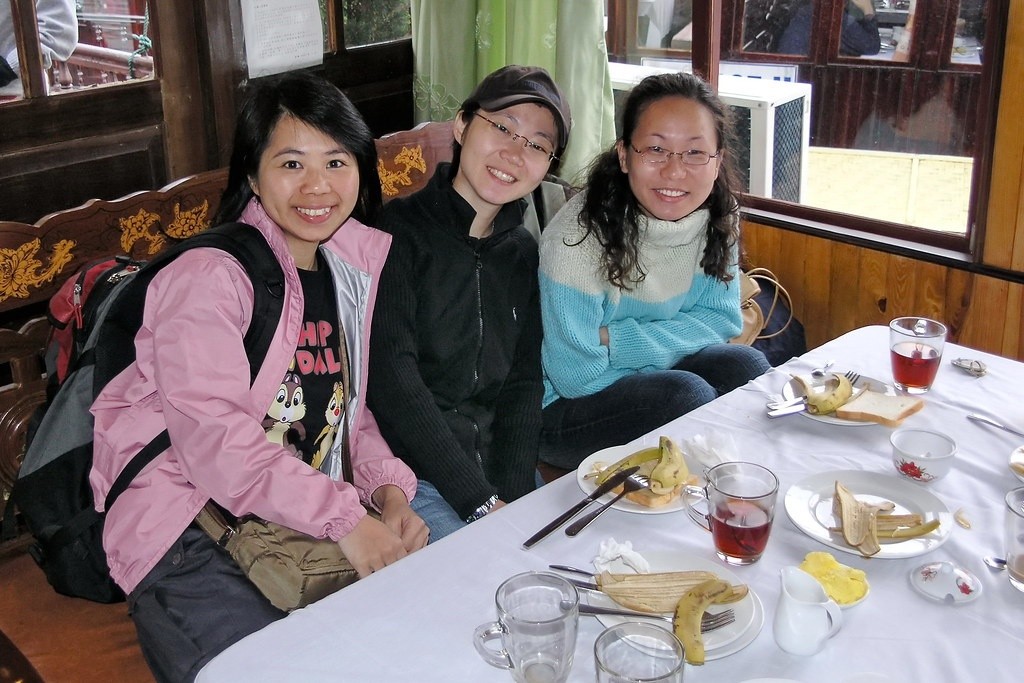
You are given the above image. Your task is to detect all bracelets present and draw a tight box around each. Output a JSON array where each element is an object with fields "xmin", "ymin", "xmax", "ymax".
[{"xmin": 465, "ymin": 493, "xmax": 499, "ymax": 524}]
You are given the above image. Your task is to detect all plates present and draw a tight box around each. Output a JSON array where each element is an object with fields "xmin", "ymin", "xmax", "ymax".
[
  {"xmin": 1008, "ymin": 445, "xmax": 1024, "ymax": 484},
  {"xmin": 783, "ymin": 469, "xmax": 953, "ymax": 560},
  {"xmin": 782, "ymin": 372, "xmax": 905, "ymax": 427},
  {"xmin": 838, "ymin": 562, "xmax": 870, "ymax": 609},
  {"xmin": 614, "ymin": 586, "xmax": 765, "ymax": 661},
  {"xmin": 577, "ymin": 445, "xmax": 710, "ymax": 515},
  {"xmin": 587, "ymin": 549, "xmax": 755, "ymax": 652}
]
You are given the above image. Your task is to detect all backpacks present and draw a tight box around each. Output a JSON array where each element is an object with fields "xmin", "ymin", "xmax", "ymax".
[{"xmin": 13, "ymin": 222, "xmax": 286, "ymax": 603}]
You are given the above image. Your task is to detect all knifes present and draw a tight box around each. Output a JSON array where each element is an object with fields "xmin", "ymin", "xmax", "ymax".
[{"xmin": 523, "ymin": 465, "xmax": 641, "ymax": 550}]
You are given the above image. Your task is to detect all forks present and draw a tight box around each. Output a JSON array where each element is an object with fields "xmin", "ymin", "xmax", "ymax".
[
  {"xmin": 560, "ymin": 600, "xmax": 735, "ymax": 634},
  {"xmin": 565, "ymin": 473, "xmax": 650, "ymax": 537},
  {"xmin": 765, "ymin": 371, "xmax": 860, "ymax": 411}
]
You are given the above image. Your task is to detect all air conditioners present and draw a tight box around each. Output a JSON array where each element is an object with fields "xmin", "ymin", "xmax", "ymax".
[{"xmin": 607, "ymin": 62, "xmax": 811, "ymax": 204}]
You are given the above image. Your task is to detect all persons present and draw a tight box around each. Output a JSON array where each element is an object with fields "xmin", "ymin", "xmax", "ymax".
[
  {"xmin": 954, "ymin": 2, "xmax": 989, "ymax": 46},
  {"xmin": 89, "ymin": 74, "xmax": 430, "ymax": 683},
  {"xmin": 539, "ymin": 72, "xmax": 772, "ymax": 472},
  {"xmin": 366, "ymin": 64, "xmax": 571, "ymax": 547},
  {"xmin": 0, "ymin": 0, "xmax": 78, "ymax": 96},
  {"xmin": 776, "ymin": 0, "xmax": 881, "ymax": 57}
]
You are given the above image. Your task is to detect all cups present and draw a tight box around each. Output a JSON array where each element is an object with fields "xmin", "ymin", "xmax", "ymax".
[
  {"xmin": 889, "ymin": 316, "xmax": 948, "ymax": 395},
  {"xmin": 679, "ymin": 461, "xmax": 780, "ymax": 567},
  {"xmin": 473, "ymin": 570, "xmax": 581, "ymax": 683},
  {"xmin": 594, "ymin": 621, "xmax": 685, "ymax": 683},
  {"xmin": 1004, "ymin": 486, "xmax": 1024, "ymax": 593}
]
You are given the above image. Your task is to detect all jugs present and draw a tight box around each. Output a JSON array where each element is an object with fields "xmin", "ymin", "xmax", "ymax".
[{"xmin": 772, "ymin": 565, "xmax": 843, "ymax": 658}]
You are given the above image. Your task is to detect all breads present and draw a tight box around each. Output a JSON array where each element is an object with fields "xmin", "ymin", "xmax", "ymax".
[
  {"xmin": 835, "ymin": 390, "xmax": 924, "ymax": 427},
  {"xmin": 604, "ymin": 459, "xmax": 699, "ymax": 508}
]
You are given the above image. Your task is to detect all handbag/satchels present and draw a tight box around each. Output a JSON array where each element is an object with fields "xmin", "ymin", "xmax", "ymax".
[{"xmin": 230, "ymin": 508, "xmax": 384, "ymax": 612}]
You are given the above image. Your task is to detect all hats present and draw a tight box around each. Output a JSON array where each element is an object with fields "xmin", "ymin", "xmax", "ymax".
[{"xmin": 471, "ymin": 66, "xmax": 572, "ymax": 151}]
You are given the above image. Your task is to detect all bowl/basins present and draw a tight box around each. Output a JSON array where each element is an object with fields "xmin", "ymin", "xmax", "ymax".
[{"xmin": 889, "ymin": 427, "xmax": 957, "ymax": 485}]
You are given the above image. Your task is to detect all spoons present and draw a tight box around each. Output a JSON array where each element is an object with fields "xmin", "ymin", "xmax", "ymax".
[{"xmin": 809, "ymin": 361, "xmax": 834, "ymax": 377}]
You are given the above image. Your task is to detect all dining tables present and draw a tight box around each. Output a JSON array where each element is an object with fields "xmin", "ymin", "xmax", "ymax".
[{"xmin": 191, "ymin": 324, "xmax": 1024, "ymax": 683}]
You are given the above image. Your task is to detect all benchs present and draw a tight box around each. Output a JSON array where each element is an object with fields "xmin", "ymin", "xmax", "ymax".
[{"xmin": 0, "ymin": 124, "xmax": 578, "ymax": 683}]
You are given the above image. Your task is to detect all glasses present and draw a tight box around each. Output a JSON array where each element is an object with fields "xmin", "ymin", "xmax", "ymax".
[
  {"xmin": 628, "ymin": 140, "xmax": 719, "ymax": 165},
  {"xmin": 473, "ymin": 112, "xmax": 563, "ymax": 165}
]
